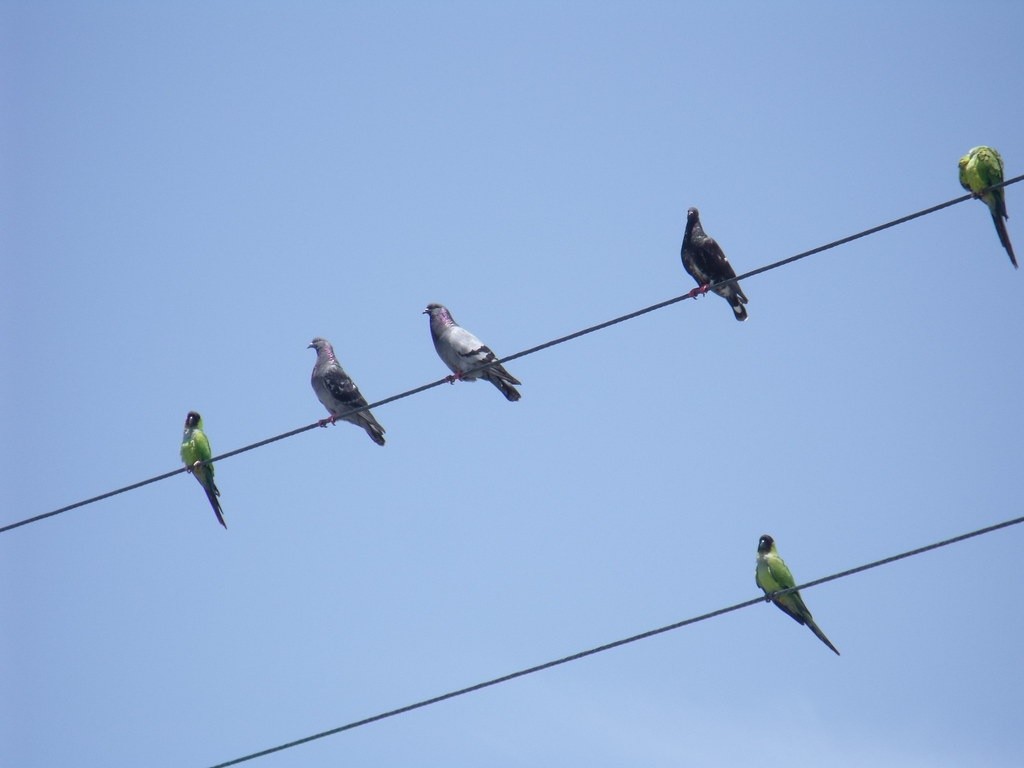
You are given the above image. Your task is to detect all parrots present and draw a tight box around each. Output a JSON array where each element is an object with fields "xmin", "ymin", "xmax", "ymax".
[
  {"xmin": 180, "ymin": 411, "xmax": 227, "ymax": 530},
  {"xmin": 755, "ymin": 536, "xmax": 839, "ymax": 656}
]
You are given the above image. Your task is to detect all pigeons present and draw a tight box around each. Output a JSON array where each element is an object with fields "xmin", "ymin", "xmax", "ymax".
[
  {"xmin": 422, "ymin": 303, "xmax": 522, "ymax": 402},
  {"xmin": 308, "ymin": 337, "xmax": 387, "ymax": 447},
  {"xmin": 680, "ymin": 207, "xmax": 748, "ymax": 322}
]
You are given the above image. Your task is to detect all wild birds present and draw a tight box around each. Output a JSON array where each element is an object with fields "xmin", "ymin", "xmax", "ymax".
[{"xmin": 958, "ymin": 145, "xmax": 1019, "ymax": 270}]
